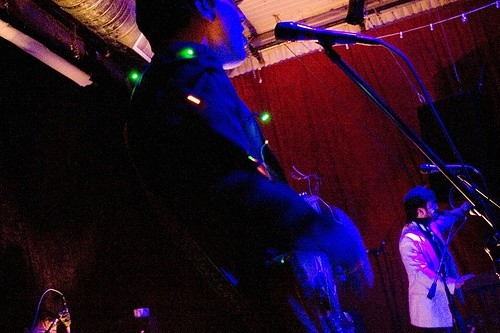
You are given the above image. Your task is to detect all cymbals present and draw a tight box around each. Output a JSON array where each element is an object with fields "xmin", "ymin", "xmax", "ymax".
[{"xmin": 454, "ymin": 271, "xmax": 494, "ymax": 296}]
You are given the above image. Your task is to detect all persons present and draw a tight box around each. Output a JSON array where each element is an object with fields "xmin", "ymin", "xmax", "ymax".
[
  {"xmin": 398, "ymin": 186, "xmax": 478, "ymax": 332},
  {"xmin": 128, "ymin": 0, "xmax": 377, "ymax": 333}
]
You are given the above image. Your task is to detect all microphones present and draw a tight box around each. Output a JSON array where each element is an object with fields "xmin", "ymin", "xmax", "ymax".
[
  {"xmin": 418, "ymin": 164, "xmax": 479, "ymax": 175},
  {"xmin": 377, "ymin": 239, "xmax": 386, "ymax": 257},
  {"xmin": 273, "ymin": 22, "xmax": 383, "ymax": 46}
]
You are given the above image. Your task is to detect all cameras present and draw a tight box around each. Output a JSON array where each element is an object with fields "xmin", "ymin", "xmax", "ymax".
[{"xmin": 131, "ymin": 304, "xmax": 151, "ymax": 320}]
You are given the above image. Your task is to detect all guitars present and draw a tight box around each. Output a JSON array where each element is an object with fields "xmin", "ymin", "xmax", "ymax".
[{"xmin": 267, "ymin": 168, "xmax": 373, "ymax": 332}]
